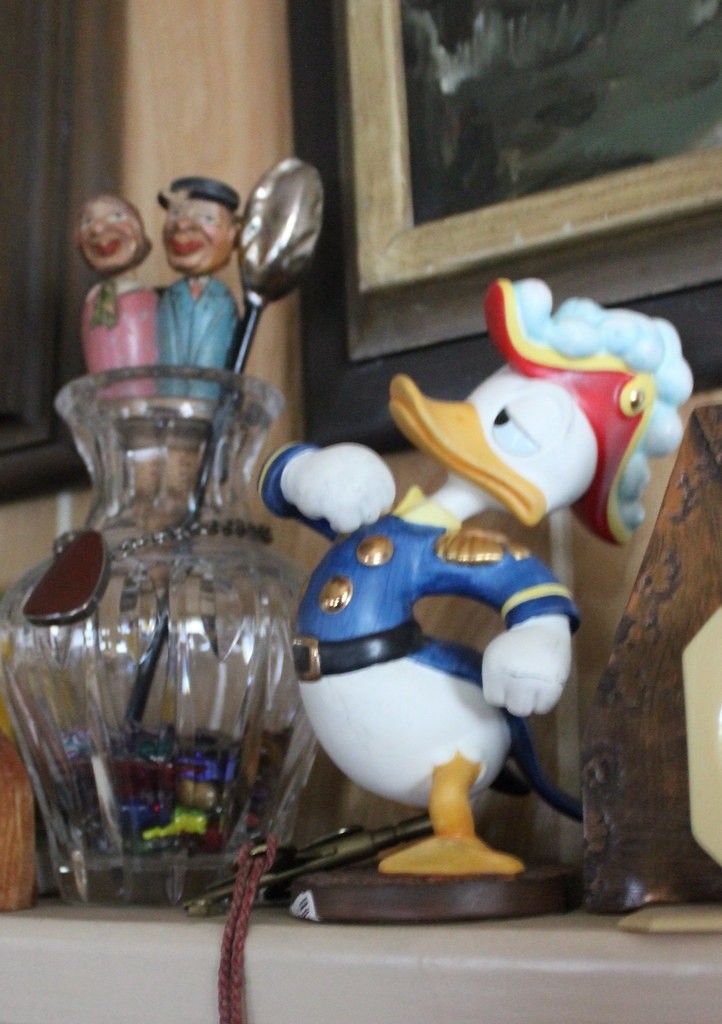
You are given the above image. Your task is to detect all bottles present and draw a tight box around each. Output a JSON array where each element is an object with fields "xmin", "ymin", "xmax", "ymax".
[{"xmin": 0, "ymin": 365, "xmax": 318, "ymax": 914}]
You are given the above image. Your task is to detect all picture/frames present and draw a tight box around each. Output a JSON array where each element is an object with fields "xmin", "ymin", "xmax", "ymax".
[
  {"xmin": 285, "ymin": 2, "xmax": 722, "ymax": 459},
  {"xmin": 0, "ymin": 1, "xmax": 130, "ymax": 511}
]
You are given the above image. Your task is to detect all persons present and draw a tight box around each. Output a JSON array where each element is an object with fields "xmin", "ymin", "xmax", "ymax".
[
  {"xmin": 155, "ymin": 175, "xmax": 243, "ymax": 398},
  {"xmin": 69, "ymin": 193, "xmax": 159, "ymax": 402}
]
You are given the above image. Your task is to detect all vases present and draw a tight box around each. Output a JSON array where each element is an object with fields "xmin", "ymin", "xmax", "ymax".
[{"xmin": 1, "ymin": 365, "xmax": 319, "ymax": 906}]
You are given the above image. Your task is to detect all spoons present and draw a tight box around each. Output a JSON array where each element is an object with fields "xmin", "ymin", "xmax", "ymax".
[{"xmin": 122, "ymin": 155, "xmax": 325, "ymax": 735}]
